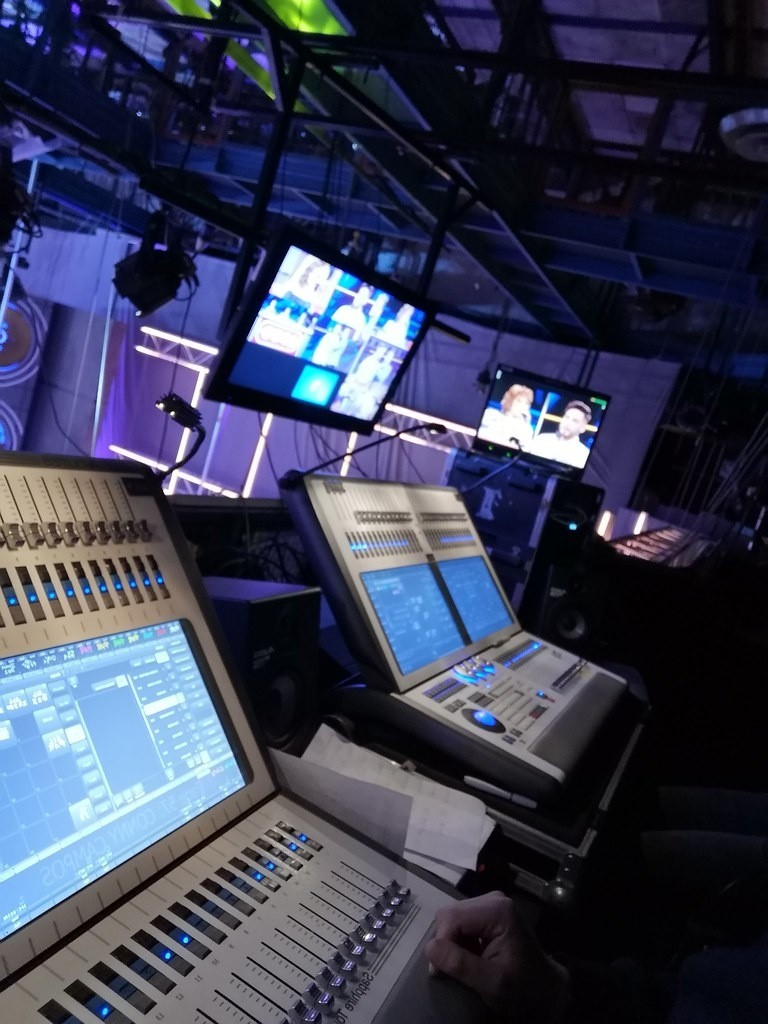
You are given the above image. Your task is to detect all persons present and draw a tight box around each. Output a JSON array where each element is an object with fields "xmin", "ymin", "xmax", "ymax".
[
  {"xmin": 480, "ymin": 384, "xmax": 590, "ymax": 467},
  {"xmin": 249, "ymin": 250, "xmax": 416, "ymax": 424},
  {"xmin": 426, "ymin": 778, "xmax": 768, "ymax": 1024}
]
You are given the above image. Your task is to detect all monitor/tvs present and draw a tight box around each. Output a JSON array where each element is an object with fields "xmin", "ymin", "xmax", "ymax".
[
  {"xmin": 472, "ymin": 363, "xmax": 612, "ymax": 481},
  {"xmin": 360, "ymin": 563, "xmax": 467, "ymax": 676},
  {"xmin": 0, "ymin": 620, "xmax": 246, "ymax": 939},
  {"xmin": 204, "ymin": 225, "xmax": 440, "ymax": 436},
  {"xmin": 436, "ymin": 555, "xmax": 513, "ymax": 643}
]
